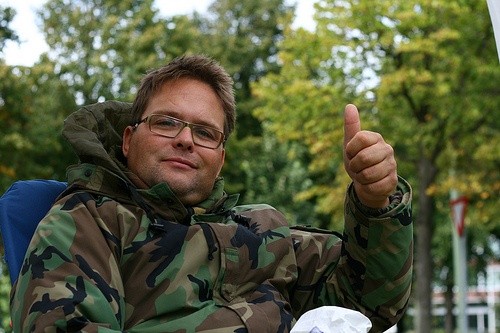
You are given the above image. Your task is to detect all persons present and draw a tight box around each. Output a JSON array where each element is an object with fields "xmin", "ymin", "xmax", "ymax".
[{"xmin": 9, "ymin": 56, "xmax": 413, "ymax": 333}]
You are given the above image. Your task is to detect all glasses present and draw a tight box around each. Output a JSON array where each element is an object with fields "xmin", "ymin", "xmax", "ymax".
[{"xmin": 131, "ymin": 112, "xmax": 226, "ymax": 150}]
